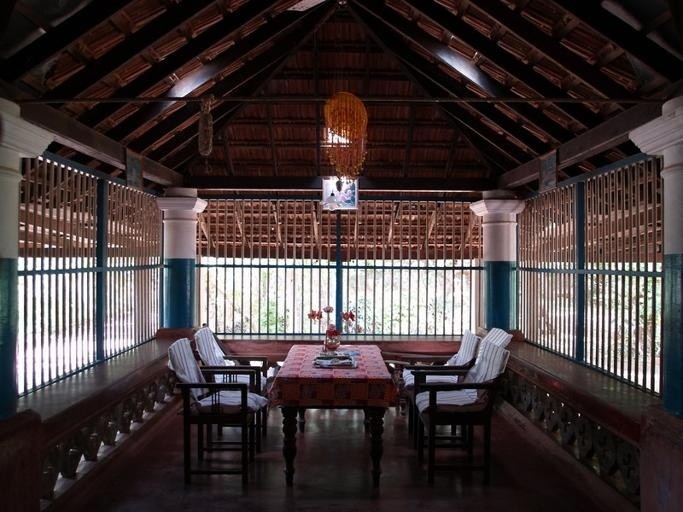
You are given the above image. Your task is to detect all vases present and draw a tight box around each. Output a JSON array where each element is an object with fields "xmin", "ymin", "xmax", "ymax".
[{"xmin": 322, "ymin": 334, "xmax": 341, "ymax": 349}]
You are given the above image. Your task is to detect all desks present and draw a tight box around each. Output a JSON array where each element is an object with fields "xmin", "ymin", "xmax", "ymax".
[{"xmin": 264, "ymin": 338, "xmax": 399, "ymax": 489}]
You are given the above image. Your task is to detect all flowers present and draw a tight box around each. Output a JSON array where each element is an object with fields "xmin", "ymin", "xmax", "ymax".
[{"xmin": 306, "ymin": 303, "xmax": 355, "ymax": 341}]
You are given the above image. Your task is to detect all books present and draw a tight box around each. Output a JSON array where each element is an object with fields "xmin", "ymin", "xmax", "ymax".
[{"xmin": 316, "ymin": 351, "xmax": 351, "ymax": 360}]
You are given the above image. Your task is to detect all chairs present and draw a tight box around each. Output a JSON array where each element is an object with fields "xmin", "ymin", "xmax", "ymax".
[
  {"xmin": 391, "ymin": 326, "xmax": 480, "ymax": 451},
  {"xmin": 406, "ymin": 337, "xmax": 518, "ymax": 491},
  {"xmin": 189, "ymin": 323, "xmax": 274, "ymax": 442},
  {"xmin": 382, "ymin": 322, "xmax": 516, "ymax": 416},
  {"xmin": 164, "ymin": 335, "xmax": 271, "ymax": 494}
]
